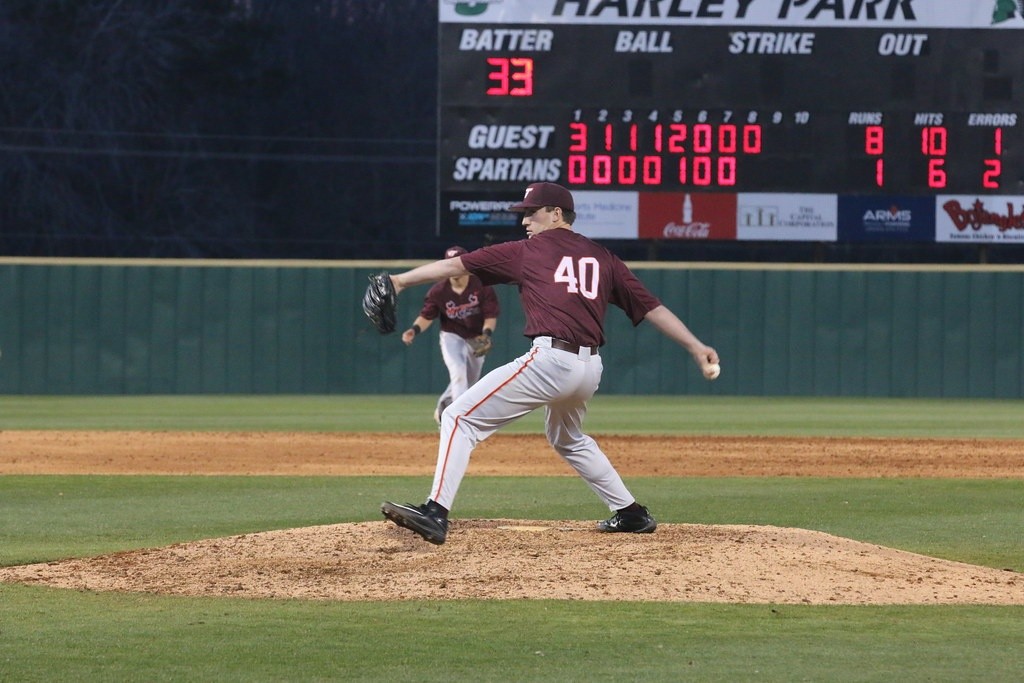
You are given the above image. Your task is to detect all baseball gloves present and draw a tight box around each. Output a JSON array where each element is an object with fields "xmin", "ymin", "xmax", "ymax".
[
  {"xmin": 474, "ymin": 335, "xmax": 490, "ymax": 358},
  {"xmin": 362, "ymin": 271, "xmax": 398, "ymax": 338}
]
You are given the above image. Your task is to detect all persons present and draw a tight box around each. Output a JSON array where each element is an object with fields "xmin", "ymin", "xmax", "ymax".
[
  {"xmin": 360, "ymin": 179, "xmax": 723, "ymax": 547},
  {"xmin": 403, "ymin": 244, "xmax": 500, "ymax": 435}
]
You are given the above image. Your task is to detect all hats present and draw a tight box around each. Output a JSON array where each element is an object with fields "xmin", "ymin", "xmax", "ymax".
[
  {"xmin": 508, "ymin": 182, "xmax": 574, "ymax": 212},
  {"xmin": 445, "ymin": 246, "xmax": 468, "ymax": 260}
]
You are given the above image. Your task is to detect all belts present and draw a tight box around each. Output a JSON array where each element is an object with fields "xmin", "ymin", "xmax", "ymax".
[{"xmin": 552, "ymin": 338, "xmax": 598, "ymax": 355}]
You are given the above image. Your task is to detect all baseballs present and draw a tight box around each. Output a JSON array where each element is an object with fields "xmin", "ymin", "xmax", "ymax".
[{"xmin": 707, "ymin": 362, "xmax": 721, "ymax": 382}]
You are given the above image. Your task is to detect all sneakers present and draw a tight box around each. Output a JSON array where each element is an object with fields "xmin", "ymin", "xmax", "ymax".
[
  {"xmin": 379, "ymin": 500, "xmax": 447, "ymax": 544},
  {"xmin": 597, "ymin": 506, "xmax": 656, "ymax": 533}
]
